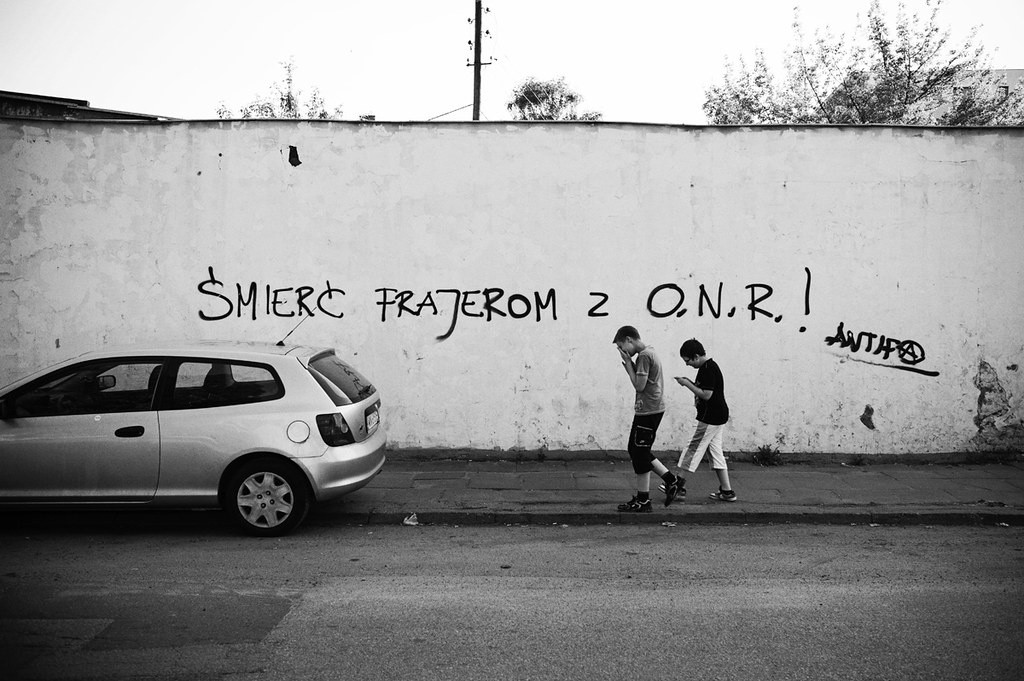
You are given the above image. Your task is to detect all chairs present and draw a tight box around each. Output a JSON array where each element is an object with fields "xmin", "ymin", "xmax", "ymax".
[
  {"xmin": 199, "ymin": 363, "xmax": 236, "ymax": 407},
  {"xmin": 146, "ymin": 364, "xmax": 162, "ymax": 396}
]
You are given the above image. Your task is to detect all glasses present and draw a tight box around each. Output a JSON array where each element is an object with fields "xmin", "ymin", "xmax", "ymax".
[{"xmin": 684, "ymin": 358, "xmax": 691, "ymax": 363}]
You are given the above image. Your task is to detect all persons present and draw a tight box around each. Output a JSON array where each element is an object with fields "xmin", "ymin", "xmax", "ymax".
[
  {"xmin": 612, "ymin": 326, "xmax": 686, "ymax": 511},
  {"xmin": 657, "ymin": 340, "xmax": 737, "ymax": 502}
]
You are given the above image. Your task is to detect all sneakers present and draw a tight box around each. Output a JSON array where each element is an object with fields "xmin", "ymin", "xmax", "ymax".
[
  {"xmin": 617, "ymin": 495, "xmax": 654, "ymax": 513},
  {"xmin": 662, "ymin": 475, "xmax": 688, "ymax": 507},
  {"xmin": 707, "ymin": 486, "xmax": 739, "ymax": 502},
  {"xmin": 658, "ymin": 483, "xmax": 688, "ymax": 500}
]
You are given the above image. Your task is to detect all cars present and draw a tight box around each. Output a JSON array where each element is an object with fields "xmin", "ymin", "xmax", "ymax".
[{"xmin": 1, "ymin": 340, "xmax": 387, "ymax": 537}]
